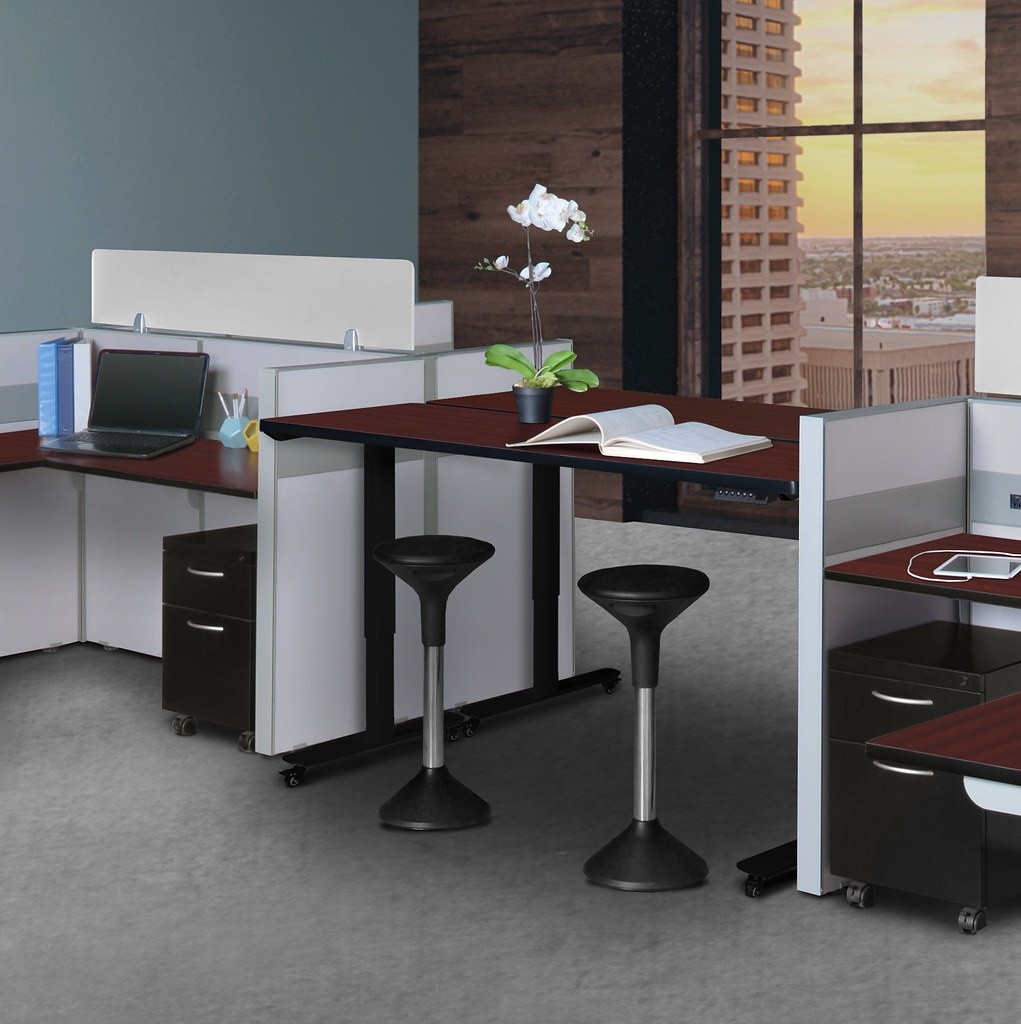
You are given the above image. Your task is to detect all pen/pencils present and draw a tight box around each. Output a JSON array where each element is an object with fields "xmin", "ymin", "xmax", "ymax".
[{"xmin": 217, "ymin": 388, "xmax": 248, "ymax": 419}]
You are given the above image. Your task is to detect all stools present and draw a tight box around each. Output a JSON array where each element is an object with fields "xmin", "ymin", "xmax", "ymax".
[
  {"xmin": 371, "ymin": 535, "xmax": 496, "ymax": 832},
  {"xmin": 577, "ymin": 565, "xmax": 710, "ymax": 892}
]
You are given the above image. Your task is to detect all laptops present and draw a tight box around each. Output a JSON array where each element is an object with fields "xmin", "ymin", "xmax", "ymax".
[{"xmin": 40, "ymin": 349, "xmax": 211, "ymax": 458}]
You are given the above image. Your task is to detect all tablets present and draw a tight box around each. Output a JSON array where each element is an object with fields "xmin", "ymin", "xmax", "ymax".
[{"xmin": 933, "ymin": 554, "xmax": 1021, "ymax": 579}]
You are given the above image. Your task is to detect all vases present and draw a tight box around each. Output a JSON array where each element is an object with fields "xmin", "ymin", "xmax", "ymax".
[{"xmin": 512, "ymin": 384, "xmax": 555, "ymax": 423}]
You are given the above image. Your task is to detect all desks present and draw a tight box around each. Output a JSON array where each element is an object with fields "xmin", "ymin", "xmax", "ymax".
[
  {"xmin": 1, "ymin": 428, "xmax": 258, "ymax": 499},
  {"xmin": 865, "ymin": 689, "xmax": 1020, "ymax": 790},
  {"xmin": 822, "ymin": 534, "xmax": 1021, "ymax": 609},
  {"xmin": 259, "ymin": 385, "xmax": 842, "ymax": 900}
]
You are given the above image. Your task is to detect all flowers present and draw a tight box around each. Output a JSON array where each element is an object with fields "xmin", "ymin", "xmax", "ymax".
[{"xmin": 474, "ymin": 183, "xmax": 600, "ymax": 390}]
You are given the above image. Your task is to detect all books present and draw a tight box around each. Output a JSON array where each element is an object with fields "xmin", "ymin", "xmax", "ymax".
[
  {"xmin": 37, "ymin": 337, "xmax": 95, "ymax": 436},
  {"xmin": 506, "ymin": 405, "xmax": 773, "ymax": 464}
]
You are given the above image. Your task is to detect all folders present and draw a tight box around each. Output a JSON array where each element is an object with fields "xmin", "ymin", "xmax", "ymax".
[{"xmin": 37, "ymin": 336, "xmax": 94, "ymax": 436}]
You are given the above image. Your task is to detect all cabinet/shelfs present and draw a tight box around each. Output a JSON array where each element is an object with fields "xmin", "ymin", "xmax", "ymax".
[
  {"xmin": 160, "ymin": 523, "xmax": 258, "ymax": 754},
  {"xmin": 825, "ymin": 619, "xmax": 1021, "ymax": 938}
]
